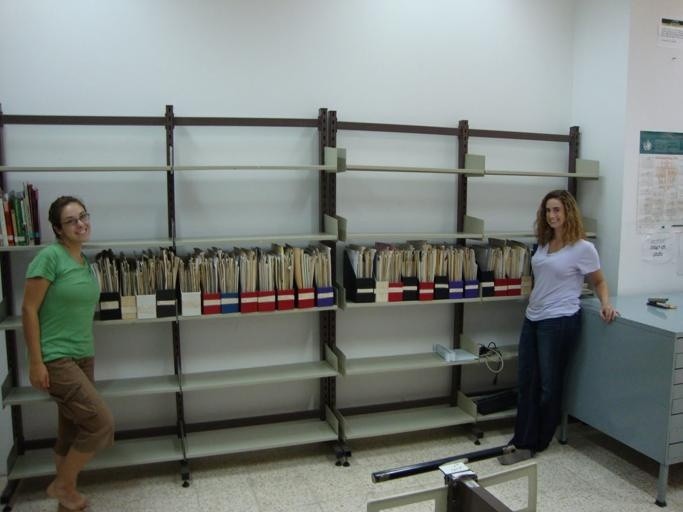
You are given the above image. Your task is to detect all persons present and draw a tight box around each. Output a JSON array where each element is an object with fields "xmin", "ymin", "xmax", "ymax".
[
  {"xmin": 498, "ymin": 189, "xmax": 622, "ymax": 465},
  {"xmin": 22, "ymin": 195, "xmax": 116, "ymax": 511}
]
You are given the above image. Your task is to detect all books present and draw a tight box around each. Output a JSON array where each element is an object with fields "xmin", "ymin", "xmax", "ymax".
[
  {"xmin": 344, "ymin": 238, "xmax": 535, "ymax": 283},
  {"xmin": 87, "ymin": 241, "xmax": 333, "ymax": 298},
  {"xmin": 0, "ymin": 181, "xmax": 41, "ymax": 246}
]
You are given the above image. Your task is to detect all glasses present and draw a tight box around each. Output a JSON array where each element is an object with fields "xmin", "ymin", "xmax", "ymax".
[{"xmin": 61, "ymin": 213, "xmax": 89, "ymax": 225}]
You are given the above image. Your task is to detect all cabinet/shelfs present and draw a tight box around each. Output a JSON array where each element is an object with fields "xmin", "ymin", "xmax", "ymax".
[
  {"xmin": 0, "ymin": 105, "xmax": 600, "ymax": 493},
  {"xmin": 558, "ymin": 293, "xmax": 683, "ymax": 508}
]
